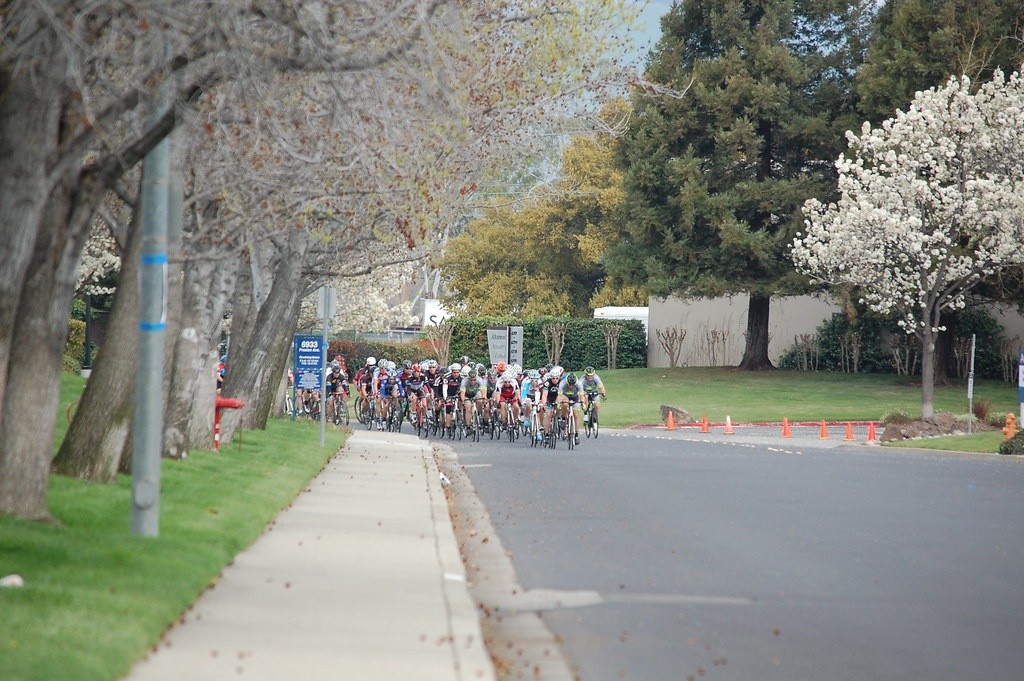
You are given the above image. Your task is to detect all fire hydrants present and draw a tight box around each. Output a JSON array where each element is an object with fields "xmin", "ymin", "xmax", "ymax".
[{"xmin": 1003, "ymin": 413, "xmax": 1021, "ymax": 442}]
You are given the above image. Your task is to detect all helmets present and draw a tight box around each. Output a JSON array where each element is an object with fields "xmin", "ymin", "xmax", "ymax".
[{"xmin": 331, "ymin": 354, "xmax": 595, "ymax": 386}]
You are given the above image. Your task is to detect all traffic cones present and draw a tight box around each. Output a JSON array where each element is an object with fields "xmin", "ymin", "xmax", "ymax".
[
  {"xmin": 819, "ymin": 420, "xmax": 830, "ymax": 438},
  {"xmin": 700, "ymin": 416, "xmax": 712, "ymax": 433},
  {"xmin": 868, "ymin": 421, "xmax": 878, "ymax": 441},
  {"xmin": 782, "ymin": 417, "xmax": 793, "ymax": 438},
  {"xmin": 666, "ymin": 410, "xmax": 678, "ymax": 430},
  {"xmin": 843, "ymin": 422, "xmax": 857, "ymax": 441},
  {"xmin": 724, "ymin": 416, "xmax": 735, "ymax": 436}
]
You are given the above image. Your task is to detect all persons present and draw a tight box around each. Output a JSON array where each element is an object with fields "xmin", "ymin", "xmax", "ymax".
[
  {"xmin": 216, "ymin": 355, "xmax": 227, "ymax": 389},
  {"xmin": 288, "ymin": 360, "xmax": 351, "ymax": 419},
  {"xmin": 353, "ymin": 356, "xmax": 607, "ymax": 446}
]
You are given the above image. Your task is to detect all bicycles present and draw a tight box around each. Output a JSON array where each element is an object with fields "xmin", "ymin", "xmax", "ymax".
[
  {"xmin": 582, "ymin": 392, "xmax": 606, "ymax": 440},
  {"xmin": 284, "ymin": 384, "xmax": 581, "ymax": 451}
]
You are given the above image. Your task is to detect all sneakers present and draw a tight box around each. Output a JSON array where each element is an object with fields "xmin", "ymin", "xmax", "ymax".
[{"xmin": 298, "ymin": 402, "xmax": 600, "ymax": 445}]
